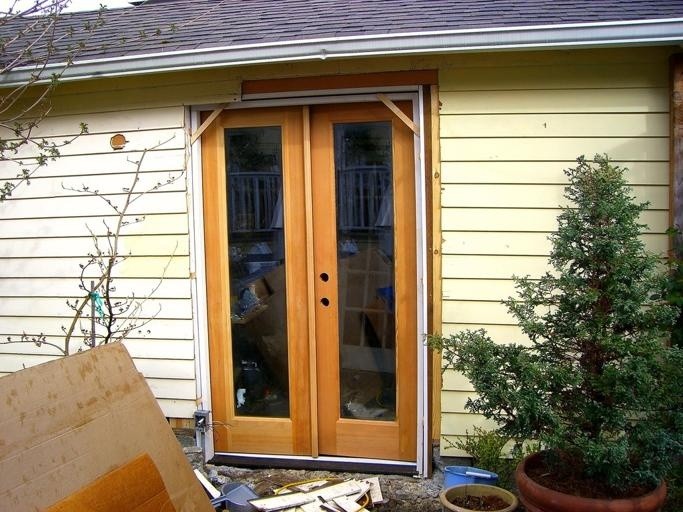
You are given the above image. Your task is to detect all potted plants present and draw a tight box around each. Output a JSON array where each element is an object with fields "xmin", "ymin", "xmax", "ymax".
[{"xmin": 421, "ymin": 151, "xmax": 683, "ymax": 512}]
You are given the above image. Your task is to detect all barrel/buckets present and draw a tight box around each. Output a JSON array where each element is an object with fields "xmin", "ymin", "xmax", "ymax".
[{"xmin": 444, "ymin": 465, "xmax": 499, "ymax": 492}]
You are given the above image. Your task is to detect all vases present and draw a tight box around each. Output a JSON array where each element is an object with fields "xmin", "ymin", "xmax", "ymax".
[{"xmin": 439, "ymin": 465, "xmax": 520, "ymax": 512}]
